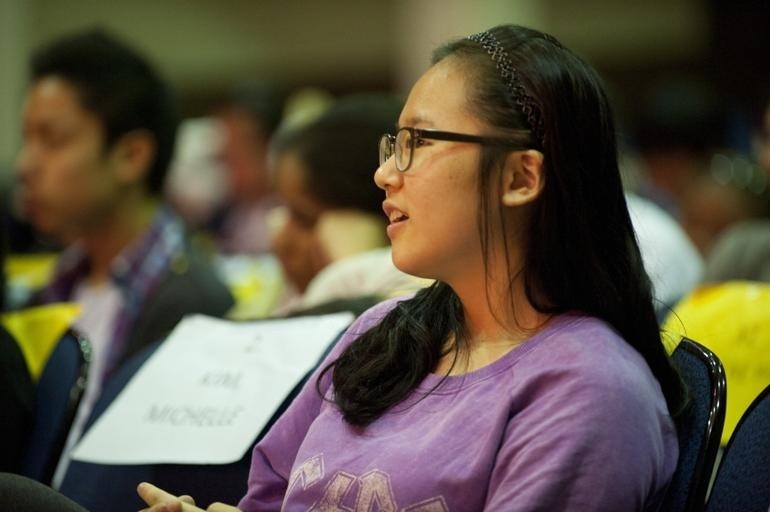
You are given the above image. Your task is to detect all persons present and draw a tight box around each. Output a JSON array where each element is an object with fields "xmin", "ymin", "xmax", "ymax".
[
  {"xmin": 1, "ymin": 33, "xmax": 235, "ymax": 369},
  {"xmin": 1, "ymin": 25, "xmax": 689, "ymax": 512},
  {"xmin": 623, "ymin": 73, "xmax": 769, "ymax": 305},
  {"xmin": 167, "ymin": 86, "xmax": 404, "ymax": 323}
]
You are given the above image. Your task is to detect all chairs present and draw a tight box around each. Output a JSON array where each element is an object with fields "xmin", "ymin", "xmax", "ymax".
[{"xmin": 1, "ymin": 325, "xmax": 769, "ymax": 512}]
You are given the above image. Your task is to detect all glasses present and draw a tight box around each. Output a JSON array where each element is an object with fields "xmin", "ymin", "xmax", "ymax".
[{"xmin": 379, "ymin": 127, "xmax": 530, "ymax": 173}]
